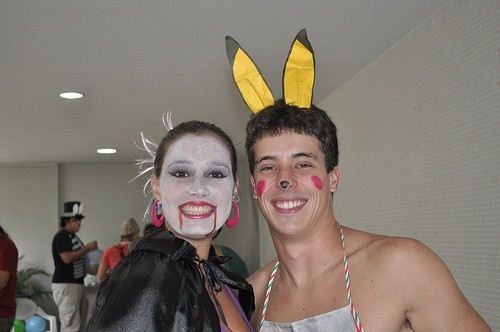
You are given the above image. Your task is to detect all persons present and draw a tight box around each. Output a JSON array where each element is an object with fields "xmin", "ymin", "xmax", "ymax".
[
  {"xmin": 51, "ymin": 202, "xmax": 99, "ymax": 332},
  {"xmin": 0, "ymin": 225, "xmax": 18, "ymax": 332},
  {"xmin": 97, "ymin": 218, "xmax": 140, "ymax": 280},
  {"xmin": 225, "ymin": 28, "xmax": 494, "ymax": 332},
  {"xmin": 144, "ymin": 223, "xmax": 162, "ymax": 238},
  {"xmin": 86, "ymin": 113, "xmax": 255, "ymax": 332}
]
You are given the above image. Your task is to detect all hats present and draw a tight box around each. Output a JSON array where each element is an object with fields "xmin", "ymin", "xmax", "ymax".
[
  {"xmin": 60, "ymin": 201, "xmax": 84, "ymax": 219},
  {"xmin": 120, "ymin": 218, "xmax": 140, "ymax": 236}
]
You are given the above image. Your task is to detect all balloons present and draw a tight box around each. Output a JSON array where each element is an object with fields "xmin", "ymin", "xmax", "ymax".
[
  {"xmin": 25, "ymin": 315, "xmax": 46, "ymax": 332},
  {"xmin": 14, "ymin": 320, "xmax": 25, "ymax": 332}
]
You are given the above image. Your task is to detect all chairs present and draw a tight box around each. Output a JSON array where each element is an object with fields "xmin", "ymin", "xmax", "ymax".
[{"xmin": 10, "ymin": 298, "xmax": 57, "ymax": 332}]
